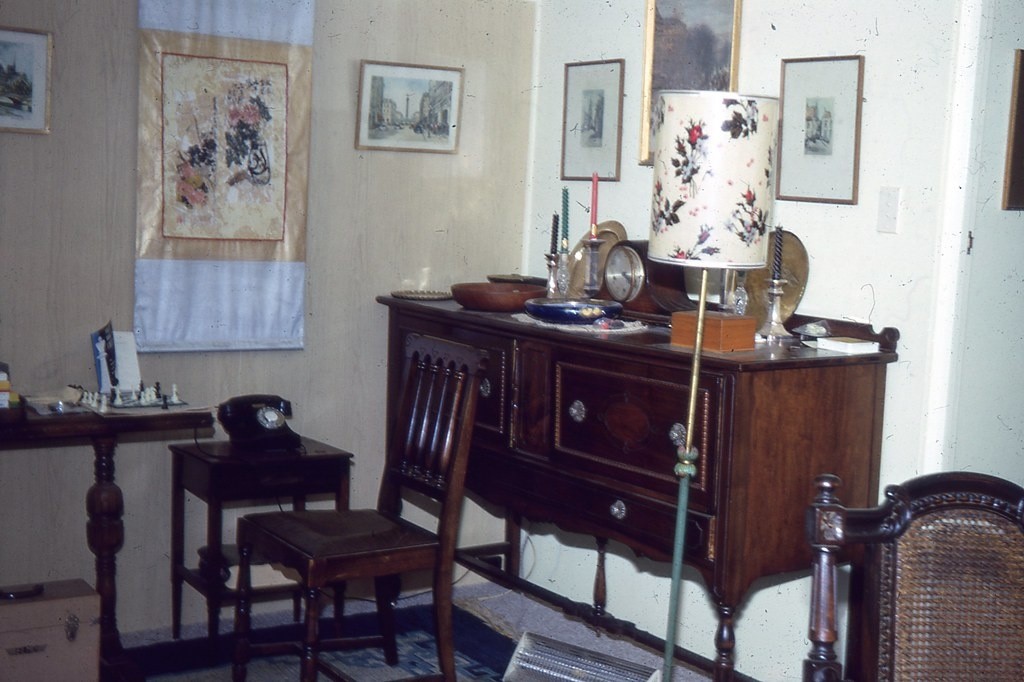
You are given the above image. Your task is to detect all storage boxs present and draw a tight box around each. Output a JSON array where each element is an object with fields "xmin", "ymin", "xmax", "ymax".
[
  {"xmin": 0, "ymin": 577, "xmax": 102, "ymax": 682},
  {"xmin": 670, "ymin": 310, "xmax": 755, "ymax": 353}
]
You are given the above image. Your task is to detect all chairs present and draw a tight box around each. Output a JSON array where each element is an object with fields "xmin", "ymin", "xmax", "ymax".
[
  {"xmin": 802, "ymin": 472, "xmax": 1024, "ymax": 682},
  {"xmin": 232, "ymin": 331, "xmax": 493, "ymax": 682}
]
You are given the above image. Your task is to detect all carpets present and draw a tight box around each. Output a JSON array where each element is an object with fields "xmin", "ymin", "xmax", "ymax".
[{"xmin": 120, "ymin": 599, "xmax": 518, "ymax": 682}]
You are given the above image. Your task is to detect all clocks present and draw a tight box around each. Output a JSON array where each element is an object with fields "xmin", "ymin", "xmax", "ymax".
[{"xmin": 599, "ymin": 238, "xmax": 687, "ymax": 318}]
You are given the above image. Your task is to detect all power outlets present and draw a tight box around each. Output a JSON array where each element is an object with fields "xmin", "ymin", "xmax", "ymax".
[{"xmin": 485, "ymin": 554, "xmax": 505, "ymax": 572}]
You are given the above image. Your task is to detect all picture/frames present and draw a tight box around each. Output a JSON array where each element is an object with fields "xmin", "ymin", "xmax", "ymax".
[
  {"xmin": 354, "ymin": 59, "xmax": 465, "ymax": 154},
  {"xmin": 0, "ymin": 24, "xmax": 56, "ymax": 135},
  {"xmin": 774, "ymin": 54, "xmax": 866, "ymax": 206},
  {"xmin": 559, "ymin": 58, "xmax": 626, "ymax": 182},
  {"xmin": 639, "ymin": 0, "xmax": 746, "ymax": 166}
]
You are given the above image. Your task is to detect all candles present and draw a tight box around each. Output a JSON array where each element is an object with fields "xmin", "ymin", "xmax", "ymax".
[
  {"xmin": 550, "ymin": 210, "xmax": 560, "ymax": 254},
  {"xmin": 591, "ymin": 170, "xmax": 599, "ymax": 240},
  {"xmin": 773, "ymin": 226, "xmax": 783, "ymax": 280},
  {"xmin": 559, "ymin": 186, "xmax": 571, "ymax": 254}
]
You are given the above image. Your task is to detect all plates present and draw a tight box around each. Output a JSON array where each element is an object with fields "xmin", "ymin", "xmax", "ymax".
[{"xmin": 390, "ymin": 289, "xmax": 451, "ymax": 301}]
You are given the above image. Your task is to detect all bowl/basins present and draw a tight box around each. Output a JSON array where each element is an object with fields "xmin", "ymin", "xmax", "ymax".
[
  {"xmin": 524, "ymin": 298, "xmax": 623, "ymax": 325},
  {"xmin": 450, "ymin": 274, "xmax": 547, "ymax": 313}
]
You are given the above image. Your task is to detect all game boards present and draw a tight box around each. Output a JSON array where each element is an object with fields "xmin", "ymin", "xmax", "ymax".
[{"xmin": 92, "ymin": 391, "xmax": 188, "ymax": 408}]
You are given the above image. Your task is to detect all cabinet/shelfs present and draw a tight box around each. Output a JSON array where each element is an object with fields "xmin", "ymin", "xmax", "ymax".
[{"xmin": 375, "ymin": 294, "xmax": 899, "ymax": 682}]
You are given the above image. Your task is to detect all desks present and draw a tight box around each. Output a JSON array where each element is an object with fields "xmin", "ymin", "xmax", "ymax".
[
  {"xmin": 167, "ymin": 432, "xmax": 353, "ymax": 644},
  {"xmin": 0, "ymin": 396, "xmax": 214, "ymax": 682}
]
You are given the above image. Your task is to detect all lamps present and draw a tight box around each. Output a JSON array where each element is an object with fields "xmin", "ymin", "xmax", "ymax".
[
  {"xmin": 499, "ymin": 632, "xmax": 663, "ymax": 682},
  {"xmin": 646, "ymin": 90, "xmax": 780, "ymax": 315}
]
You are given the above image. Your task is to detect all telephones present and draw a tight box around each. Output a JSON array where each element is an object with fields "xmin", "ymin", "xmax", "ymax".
[{"xmin": 217, "ymin": 394, "xmax": 301, "ymax": 448}]
